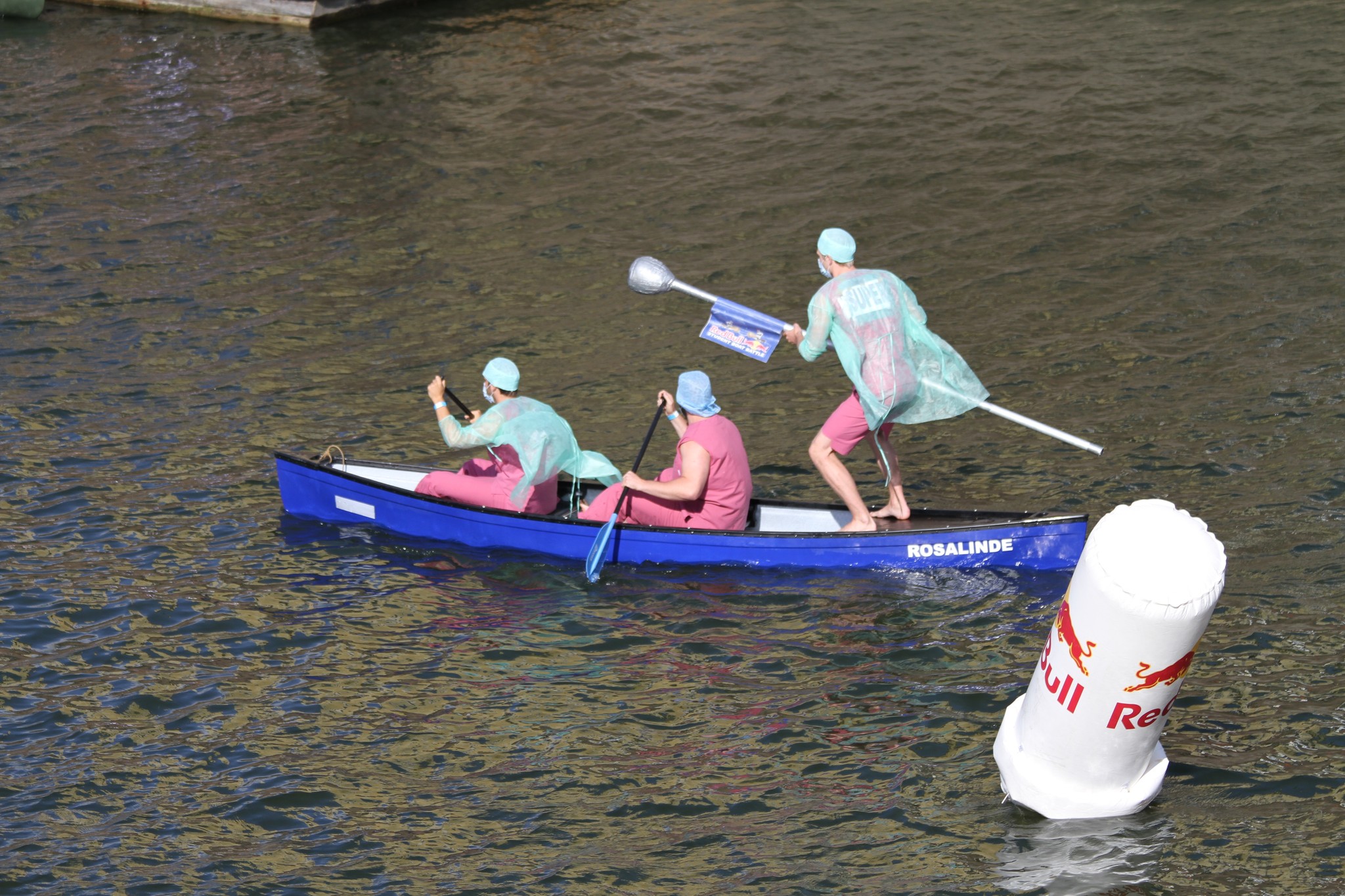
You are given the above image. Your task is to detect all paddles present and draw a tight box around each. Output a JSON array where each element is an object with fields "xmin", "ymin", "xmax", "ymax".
[
  {"xmin": 586, "ymin": 395, "xmax": 666, "ymax": 584},
  {"xmin": 441, "ymin": 376, "xmax": 474, "ymax": 419}
]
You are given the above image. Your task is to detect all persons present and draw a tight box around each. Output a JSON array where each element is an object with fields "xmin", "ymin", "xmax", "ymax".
[
  {"xmin": 783, "ymin": 229, "xmax": 991, "ymax": 533},
  {"xmin": 578, "ymin": 370, "xmax": 752, "ymax": 532},
  {"xmin": 413, "ymin": 356, "xmax": 623, "ymax": 514}
]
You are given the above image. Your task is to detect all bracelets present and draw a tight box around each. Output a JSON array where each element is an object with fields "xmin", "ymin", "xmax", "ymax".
[
  {"xmin": 664, "ymin": 410, "xmax": 680, "ymax": 420},
  {"xmin": 432, "ymin": 401, "xmax": 447, "ymax": 410}
]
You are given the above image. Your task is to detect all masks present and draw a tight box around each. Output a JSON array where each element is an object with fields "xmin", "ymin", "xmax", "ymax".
[
  {"xmin": 483, "ymin": 382, "xmax": 495, "ymax": 404},
  {"xmin": 818, "ymin": 256, "xmax": 833, "ymax": 278}
]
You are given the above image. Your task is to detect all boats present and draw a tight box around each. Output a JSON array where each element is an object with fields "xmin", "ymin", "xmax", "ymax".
[{"xmin": 273, "ymin": 447, "xmax": 1090, "ymax": 576}]
